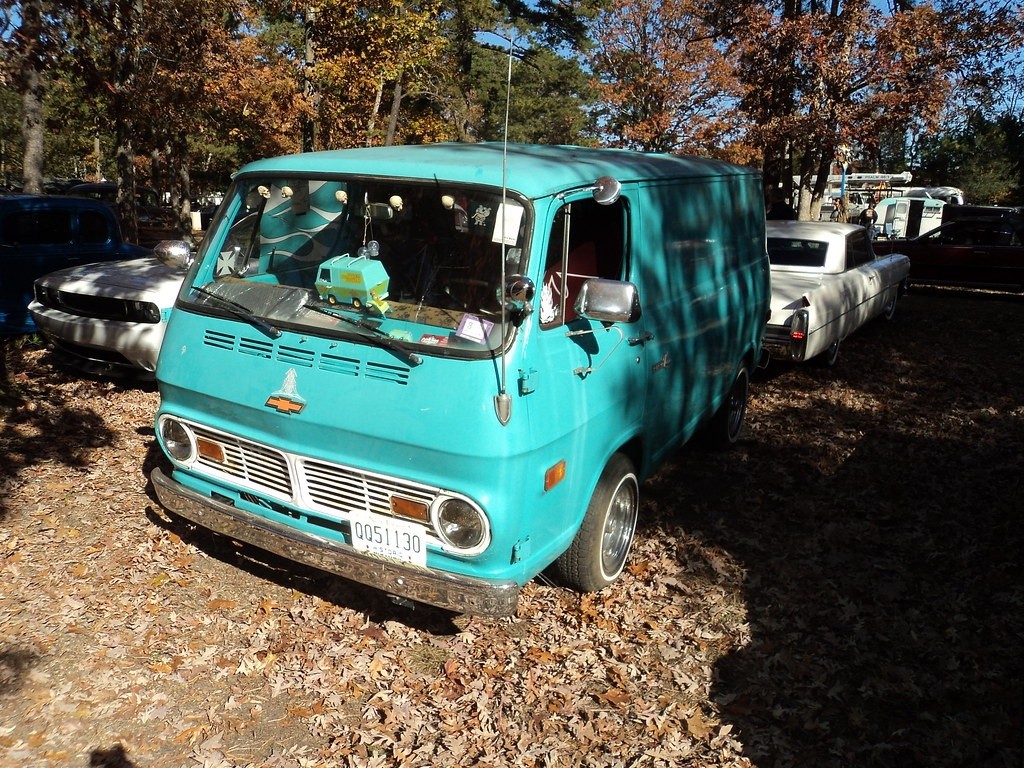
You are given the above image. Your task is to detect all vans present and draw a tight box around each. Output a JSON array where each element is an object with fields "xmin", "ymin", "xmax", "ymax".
[
  {"xmin": 872, "ymin": 197, "xmax": 947, "ymax": 239},
  {"xmin": 151, "ymin": 142, "xmax": 770, "ymax": 616}
]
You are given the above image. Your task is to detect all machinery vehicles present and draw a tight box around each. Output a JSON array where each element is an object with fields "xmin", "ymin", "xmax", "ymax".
[{"xmin": 778, "ymin": 169, "xmax": 912, "ymax": 221}]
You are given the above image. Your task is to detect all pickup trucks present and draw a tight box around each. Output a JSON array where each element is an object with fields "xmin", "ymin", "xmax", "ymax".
[{"xmin": 766, "ymin": 221, "xmax": 911, "ymax": 373}]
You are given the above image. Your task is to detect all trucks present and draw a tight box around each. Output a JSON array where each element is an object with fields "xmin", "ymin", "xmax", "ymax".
[{"xmin": 865, "ymin": 186, "xmax": 964, "ymax": 205}]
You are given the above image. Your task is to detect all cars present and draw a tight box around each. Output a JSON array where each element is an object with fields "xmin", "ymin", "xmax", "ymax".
[
  {"xmin": 0, "ymin": 170, "xmax": 261, "ymax": 247},
  {"xmin": 870, "ymin": 216, "xmax": 1024, "ymax": 293},
  {"xmin": 27, "ymin": 211, "xmax": 273, "ymax": 383},
  {"xmin": 0, "ymin": 195, "xmax": 155, "ymax": 336}
]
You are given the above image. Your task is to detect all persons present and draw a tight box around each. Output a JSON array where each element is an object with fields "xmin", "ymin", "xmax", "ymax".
[{"xmin": 858, "ymin": 194, "xmax": 878, "ymax": 228}]
